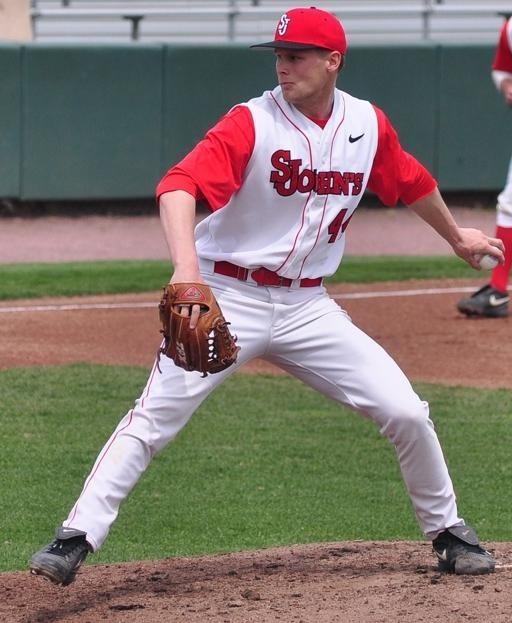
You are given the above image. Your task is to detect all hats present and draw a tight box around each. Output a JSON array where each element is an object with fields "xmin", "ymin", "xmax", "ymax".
[{"xmin": 250, "ymin": 5, "xmax": 352, "ymax": 57}]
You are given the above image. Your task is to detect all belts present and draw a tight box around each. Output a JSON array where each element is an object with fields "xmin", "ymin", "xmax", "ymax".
[{"xmin": 210, "ymin": 254, "xmax": 331, "ymax": 299}]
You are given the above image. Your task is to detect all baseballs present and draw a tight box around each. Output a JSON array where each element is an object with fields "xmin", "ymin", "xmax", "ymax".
[{"xmin": 472, "ymin": 244, "xmax": 504, "ymax": 270}]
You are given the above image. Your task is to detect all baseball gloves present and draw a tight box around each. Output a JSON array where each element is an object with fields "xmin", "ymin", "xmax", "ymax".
[{"xmin": 156, "ymin": 282, "xmax": 240, "ymax": 377}]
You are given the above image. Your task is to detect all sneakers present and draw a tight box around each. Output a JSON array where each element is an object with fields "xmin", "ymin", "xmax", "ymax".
[
  {"xmin": 432, "ymin": 521, "xmax": 497, "ymax": 576},
  {"xmin": 28, "ymin": 526, "xmax": 90, "ymax": 587},
  {"xmin": 456, "ymin": 283, "xmax": 512, "ymax": 320}
]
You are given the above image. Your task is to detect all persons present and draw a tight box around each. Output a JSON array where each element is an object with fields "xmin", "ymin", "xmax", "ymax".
[
  {"xmin": 457, "ymin": 10, "xmax": 512, "ymax": 318},
  {"xmin": 25, "ymin": 3, "xmax": 509, "ymax": 591}
]
